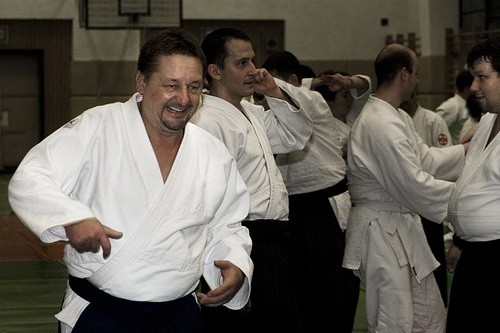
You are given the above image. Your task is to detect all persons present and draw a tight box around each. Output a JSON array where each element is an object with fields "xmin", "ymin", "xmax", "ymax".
[
  {"xmin": 8, "ymin": 28, "xmax": 254, "ymax": 333},
  {"xmin": 190, "ymin": 27, "xmax": 500, "ymax": 333}
]
